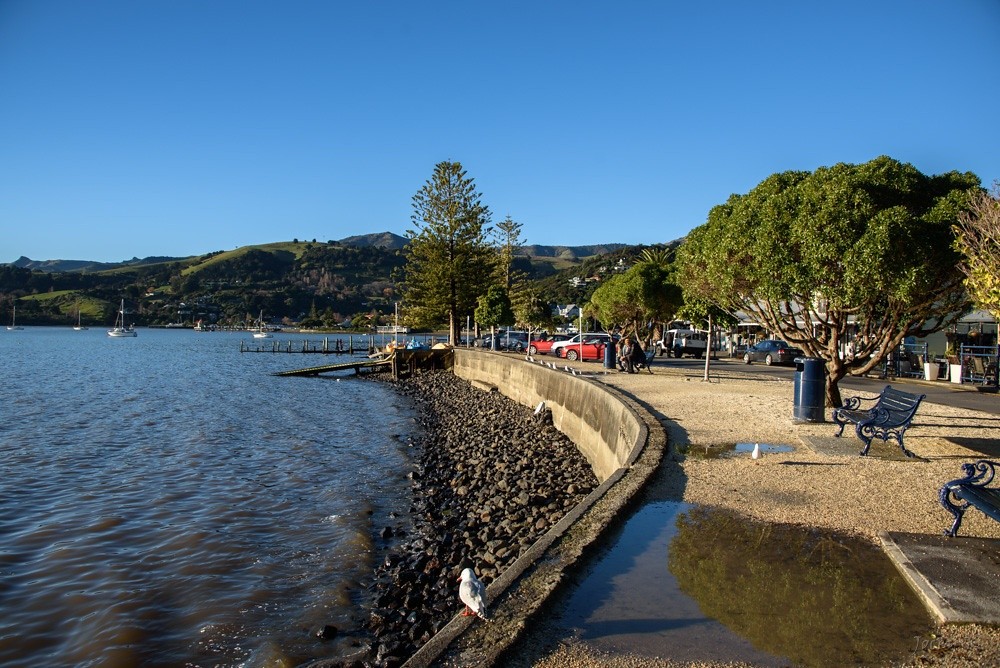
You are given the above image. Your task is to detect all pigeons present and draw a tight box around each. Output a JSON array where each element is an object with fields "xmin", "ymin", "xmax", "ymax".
[
  {"xmin": 564, "ymin": 365, "xmax": 582, "ymax": 376},
  {"xmin": 547, "ymin": 362, "xmax": 559, "ymax": 371},
  {"xmin": 456, "ymin": 568, "xmax": 487, "ymax": 619},
  {"xmin": 751, "ymin": 444, "xmax": 763, "ymax": 465},
  {"xmin": 525, "ymin": 355, "xmax": 538, "ymax": 363},
  {"xmin": 540, "ymin": 359, "xmax": 546, "ymax": 365}
]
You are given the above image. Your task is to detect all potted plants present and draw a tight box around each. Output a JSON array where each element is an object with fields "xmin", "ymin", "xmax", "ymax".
[
  {"xmin": 741, "ymin": 331, "xmax": 749, "ymax": 339},
  {"xmin": 904, "ymin": 345, "xmax": 920, "ymax": 378},
  {"xmin": 756, "ymin": 331, "xmax": 767, "ymax": 341},
  {"xmin": 924, "ymin": 351, "xmax": 940, "ymax": 381},
  {"xmin": 728, "ymin": 342, "xmax": 737, "ymax": 357},
  {"xmin": 945, "ymin": 343, "xmax": 970, "ymax": 383}
]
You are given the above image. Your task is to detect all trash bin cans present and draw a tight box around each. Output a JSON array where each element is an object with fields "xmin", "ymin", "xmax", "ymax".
[
  {"xmin": 950, "ymin": 364, "xmax": 966, "ymax": 384},
  {"xmin": 494, "ymin": 336, "xmax": 500, "ymax": 351},
  {"xmin": 923, "ymin": 363, "xmax": 940, "ymax": 380},
  {"xmin": 604, "ymin": 342, "xmax": 616, "ymax": 369},
  {"xmin": 897, "ymin": 356, "xmax": 911, "ymax": 377},
  {"xmin": 793, "ymin": 357, "xmax": 827, "ymax": 423}
]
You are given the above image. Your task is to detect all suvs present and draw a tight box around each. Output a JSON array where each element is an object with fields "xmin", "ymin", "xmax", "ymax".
[{"xmin": 653, "ymin": 329, "xmax": 706, "ymax": 360}]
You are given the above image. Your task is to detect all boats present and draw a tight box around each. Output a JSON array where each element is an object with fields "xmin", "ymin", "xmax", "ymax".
[{"xmin": 247, "ymin": 326, "xmax": 281, "ymax": 331}]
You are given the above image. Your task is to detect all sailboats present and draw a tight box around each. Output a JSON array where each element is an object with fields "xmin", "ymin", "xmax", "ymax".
[
  {"xmin": 253, "ymin": 310, "xmax": 273, "ymax": 338},
  {"xmin": 73, "ymin": 310, "xmax": 89, "ymax": 330},
  {"xmin": 107, "ymin": 299, "xmax": 137, "ymax": 337},
  {"xmin": 7, "ymin": 306, "xmax": 24, "ymax": 330}
]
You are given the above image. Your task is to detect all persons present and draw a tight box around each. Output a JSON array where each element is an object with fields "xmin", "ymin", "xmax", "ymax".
[
  {"xmin": 617, "ymin": 339, "xmax": 646, "ymax": 374},
  {"xmin": 540, "ymin": 331, "xmax": 547, "ymax": 337},
  {"xmin": 495, "ymin": 333, "xmax": 501, "ymax": 350},
  {"xmin": 336, "ymin": 338, "xmax": 343, "ymax": 350},
  {"xmin": 666, "ymin": 332, "xmax": 673, "ymax": 358}
]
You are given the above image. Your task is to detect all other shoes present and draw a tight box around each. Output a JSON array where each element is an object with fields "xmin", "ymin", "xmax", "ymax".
[{"xmin": 620, "ymin": 369, "xmax": 625, "ymax": 371}]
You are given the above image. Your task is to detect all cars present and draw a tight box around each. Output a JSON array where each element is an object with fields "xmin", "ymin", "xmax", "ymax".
[
  {"xmin": 469, "ymin": 330, "xmax": 621, "ymax": 361},
  {"xmin": 743, "ymin": 341, "xmax": 806, "ymax": 366}
]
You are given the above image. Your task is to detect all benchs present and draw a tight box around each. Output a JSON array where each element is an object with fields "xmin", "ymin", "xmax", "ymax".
[
  {"xmin": 634, "ymin": 352, "xmax": 656, "ymax": 374},
  {"xmin": 832, "ymin": 385, "xmax": 926, "ymax": 458},
  {"xmin": 508, "ymin": 344, "xmax": 525, "ymax": 354},
  {"xmin": 938, "ymin": 461, "xmax": 1000, "ymax": 538}
]
